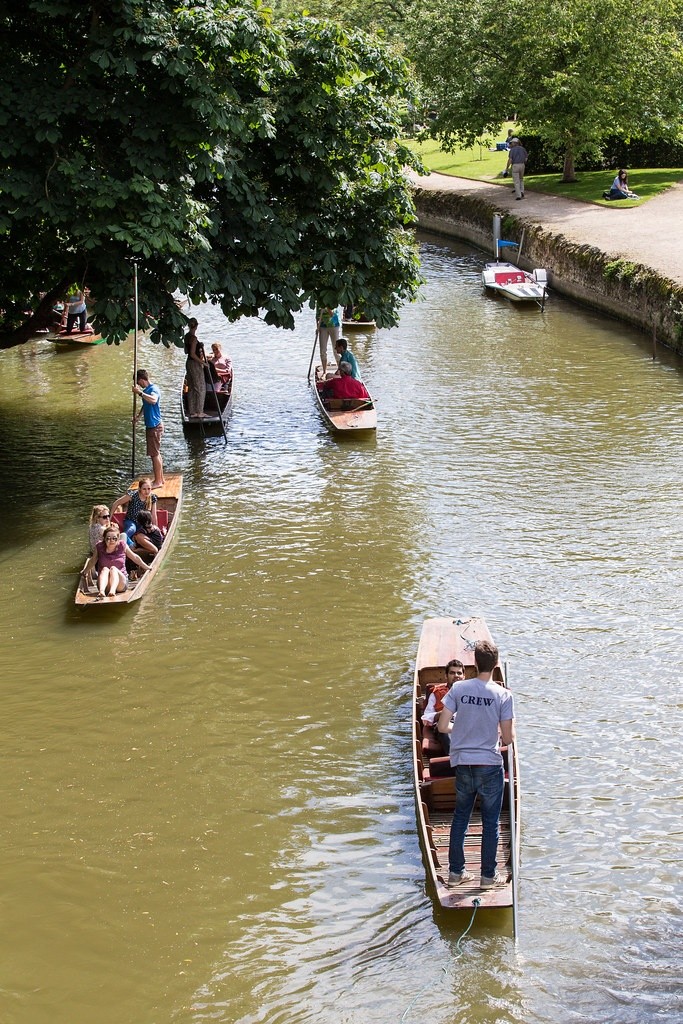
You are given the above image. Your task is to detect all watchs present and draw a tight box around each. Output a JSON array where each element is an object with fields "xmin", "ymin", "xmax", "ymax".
[{"xmin": 139, "ymin": 392, "xmax": 143, "ymax": 397}]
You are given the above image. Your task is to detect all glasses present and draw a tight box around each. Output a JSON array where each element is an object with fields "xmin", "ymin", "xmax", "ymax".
[
  {"xmin": 106, "ymin": 537, "xmax": 118, "ymax": 541},
  {"xmin": 99, "ymin": 515, "xmax": 110, "ymax": 519}
]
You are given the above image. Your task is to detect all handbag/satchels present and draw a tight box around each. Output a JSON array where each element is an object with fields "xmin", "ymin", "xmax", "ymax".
[{"xmin": 203, "ymin": 360, "xmax": 218, "ymax": 384}]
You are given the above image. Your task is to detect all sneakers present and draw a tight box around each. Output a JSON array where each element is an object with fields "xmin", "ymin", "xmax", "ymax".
[
  {"xmin": 448, "ymin": 868, "xmax": 475, "ymax": 886},
  {"xmin": 480, "ymin": 869, "xmax": 506, "ymax": 889}
]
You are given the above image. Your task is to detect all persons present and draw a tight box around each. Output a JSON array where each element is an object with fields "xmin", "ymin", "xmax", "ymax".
[
  {"xmin": 207, "ymin": 343, "xmax": 232, "ymax": 392},
  {"xmin": 124, "ymin": 510, "xmax": 163, "ymax": 580},
  {"xmin": 110, "ymin": 478, "xmax": 158, "ymax": 538},
  {"xmin": 326, "ymin": 339, "xmax": 357, "ymax": 379},
  {"xmin": 88, "ymin": 505, "xmax": 135, "ymax": 555},
  {"xmin": 131, "ymin": 369, "xmax": 164, "ymax": 489},
  {"xmin": 605, "ymin": 169, "xmax": 633, "ymax": 201},
  {"xmin": 323, "ymin": 361, "xmax": 368, "ymax": 398},
  {"xmin": 83, "ymin": 527, "xmax": 152, "ymax": 597},
  {"xmin": 506, "ymin": 137, "xmax": 528, "ymax": 200},
  {"xmin": 421, "ymin": 659, "xmax": 465, "ymax": 755},
  {"xmin": 437, "ymin": 641, "xmax": 515, "ymax": 892},
  {"xmin": 39, "ymin": 289, "xmax": 86, "ymax": 332},
  {"xmin": 183, "ymin": 318, "xmax": 210, "ymax": 418},
  {"xmin": 316, "ymin": 307, "xmax": 342, "ymax": 380}
]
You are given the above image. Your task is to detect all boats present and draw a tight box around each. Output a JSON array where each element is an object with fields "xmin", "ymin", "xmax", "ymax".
[
  {"xmin": 411, "ymin": 615, "xmax": 525, "ymax": 912},
  {"xmin": 71, "ymin": 469, "xmax": 188, "ymax": 606},
  {"xmin": 177, "ymin": 360, "xmax": 234, "ymax": 427},
  {"xmin": 29, "ymin": 297, "xmax": 191, "ymax": 346},
  {"xmin": 337, "ymin": 316, "xmax": 377, "ymax": 328},
  {"xmin": 482, "ymin": 262, "xmax": 551, "ymax": 305},
  {"xmin": 311, "ymin": 363, "xmax": 380, "ymax": 432}
]
